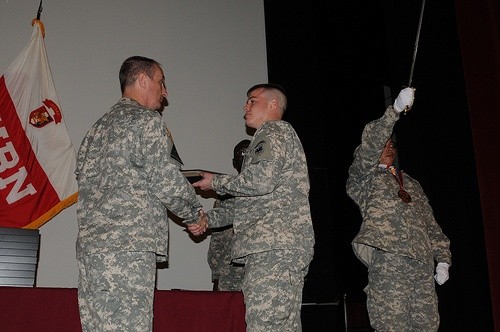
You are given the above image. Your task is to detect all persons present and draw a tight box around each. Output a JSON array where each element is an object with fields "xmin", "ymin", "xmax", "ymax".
[
  {"xmin": 346, "ymin": 86, "xmax": 451, "ymax": 332},
  {"xmin": 207, "ymin": 141, "xmax": 252, "ymax": 293},
  {"xmin": 76, "ymin": 55, "xmax": 207, "ymax": 332},
  {"xmin": 186, "ymin": 85, "xmax": 314, "ymax": 332}
]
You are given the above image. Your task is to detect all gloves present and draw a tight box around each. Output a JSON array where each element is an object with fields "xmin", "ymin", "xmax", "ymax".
[
  {"xmin": 393, "ymin": 87, "xmax": 414, "ymax": 113},
  {"xmin": 434, "ymin": 262, "xmax": 449, "ymax": 286}
]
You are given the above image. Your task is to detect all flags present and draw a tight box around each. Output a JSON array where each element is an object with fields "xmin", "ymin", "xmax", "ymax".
[{"xmin": 0, "ymin": 21, "xmax": 80, "ymax": 232}]
